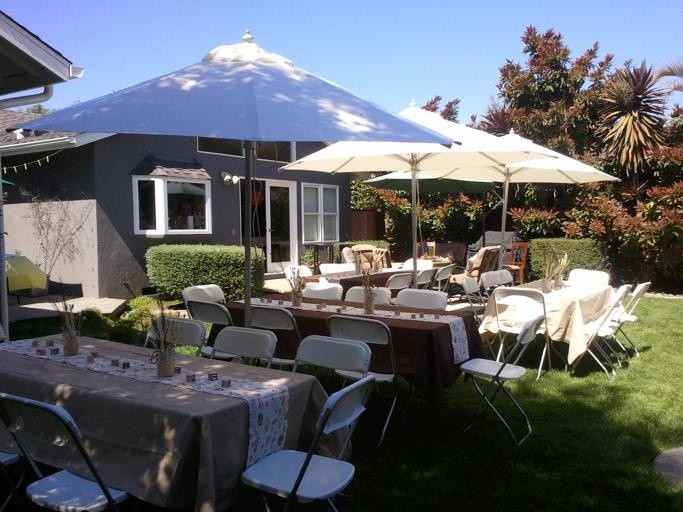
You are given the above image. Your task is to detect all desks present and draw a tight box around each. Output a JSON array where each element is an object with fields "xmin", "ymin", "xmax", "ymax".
[{"xmin": 0, "ymin": 332, "xmax": 355, "ymax": 512}]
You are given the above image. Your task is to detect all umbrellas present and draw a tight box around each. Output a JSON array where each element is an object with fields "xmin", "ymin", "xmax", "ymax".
[
  {"xmin": 5, "ymin": 27, "xmax": 464, "ymax": 329},
  {"xmin": 277, "ymin": 98, "xmax": 560, "ymax": 291},
  {"xmin": 361, "ymin": 170, "xmax": 497, "ymax": 194},
  {"xmin": 420, "ymin": 128, "xmax": 622, "ymax": 232}
]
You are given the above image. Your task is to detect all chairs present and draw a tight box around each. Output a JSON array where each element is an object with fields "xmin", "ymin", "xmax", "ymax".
[
  {"xmin": 240, "ymin": 374, "xmax": 377, "ymax": 512},
  {"xmin": 1, "ymin": 391, "xmax": 128, "ymax": 512},
  {"xmin": 143, "ymin": 316, "xmax": 206, "ymax": 358},
  {"xmin": 1, "ymin": 451, "xmax": 20, "ymax": 468}
]
